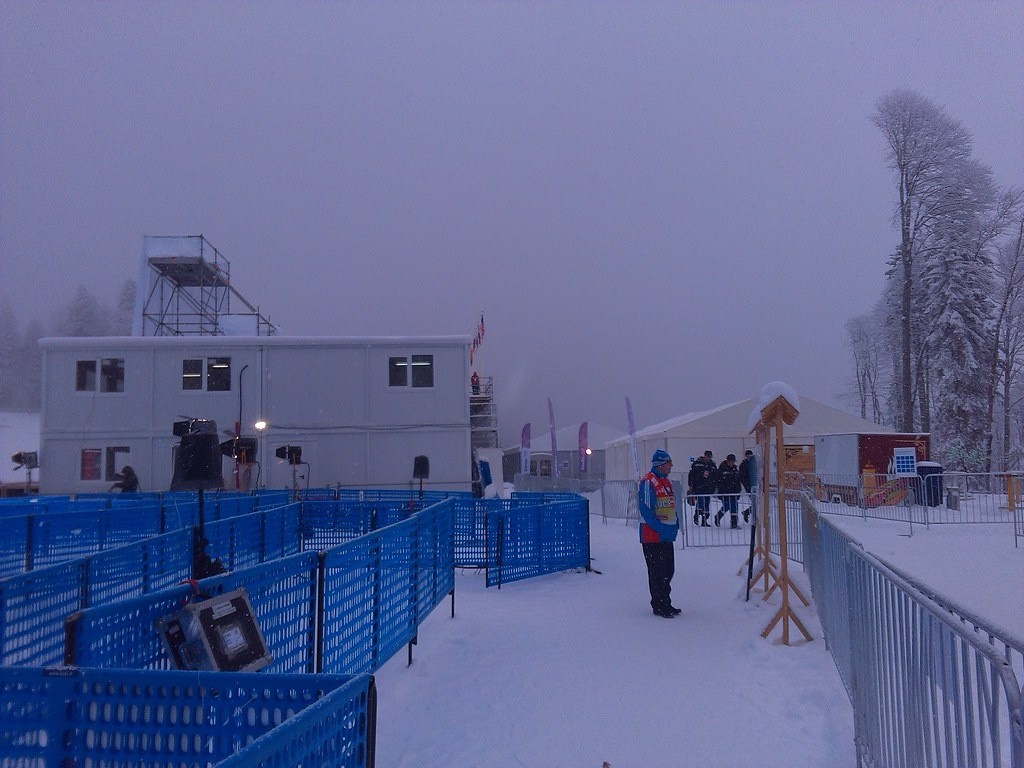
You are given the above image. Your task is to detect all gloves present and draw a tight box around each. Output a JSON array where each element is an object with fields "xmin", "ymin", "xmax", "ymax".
[
  {"xmin": 736, "ymin": 492, "xmax": 740, "ymax": 500},
  {"xmin": 717, "ymin": 492, "xmax": 723, "ymax": 500}
]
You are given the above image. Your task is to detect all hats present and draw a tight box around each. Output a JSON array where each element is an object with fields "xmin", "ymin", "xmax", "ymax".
[
  {"xmin": 727, "ymin": 454, "xmax": 736, "ymax": 461},
  {"xmin": 704, "ymin": 451, "xmax": 713, "ymax": 458},
  {"xmin": 744, "ymin": 450, "xmax": 753, "ymax": 456},
  {"xmin": 652, "ymin": 449, "xmax": 672, "ymax": 467}
]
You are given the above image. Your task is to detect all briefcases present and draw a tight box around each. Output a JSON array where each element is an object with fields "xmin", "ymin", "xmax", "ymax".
[{"xmin": 687, "ymin": 490, "xmax": 695, "ymax": 506}]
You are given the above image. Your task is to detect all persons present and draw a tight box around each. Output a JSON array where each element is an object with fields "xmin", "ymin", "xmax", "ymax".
[
  {"xmin": 111, "ymin": 466, "xmax": 138, "ymax": 492},
  {"xmin": 688, "ymin": 451, "xmax": 717, "ymax": 526},
  {"xmin": 739, "ymin": 450, "xmax": 757, "ymax": 523},
  {"xmin": 471, "ymin": 372, "xmax": 480, "ymax": 395},
  {"xmin": 639, "ymin": 450, "xmax": 682, "ymax": 619},
  {"xmin": 715, "ymin": 454, "xmax": 742, "ymax": 530}
]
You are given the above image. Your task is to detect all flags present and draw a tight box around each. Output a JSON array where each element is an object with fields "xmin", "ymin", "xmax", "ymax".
[{"xmin": 470, "ymin": 315, "xmax": 484, "ymax": 365}]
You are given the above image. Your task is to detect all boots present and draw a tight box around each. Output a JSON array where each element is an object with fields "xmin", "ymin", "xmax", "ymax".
[
  {"xmin": 701, "ymin": 511, "xmax": 711, "ymax": 527},
  {"xmin": 694, "ymin": 508, "xmax": 703, "ymax": 525},
  {"xmin": 741, "ymin": 507, "xmax": 751, "ymax": 523},
  {"xmin": 731, "ymin": 514, "xmax": 741, "ymax": 529},
  {"xmin": 714, "ymin": 509, "xmax": 724, "ymax": 527}
]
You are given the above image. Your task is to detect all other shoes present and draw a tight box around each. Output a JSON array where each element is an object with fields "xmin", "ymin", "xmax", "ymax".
[
  {"xmin": 653, "ymin": 606, "xmax": 674, "ymax": 618},
  {"xmin": 665, "ymin": 603, "xmax": 681, "ymax": 615}
]
